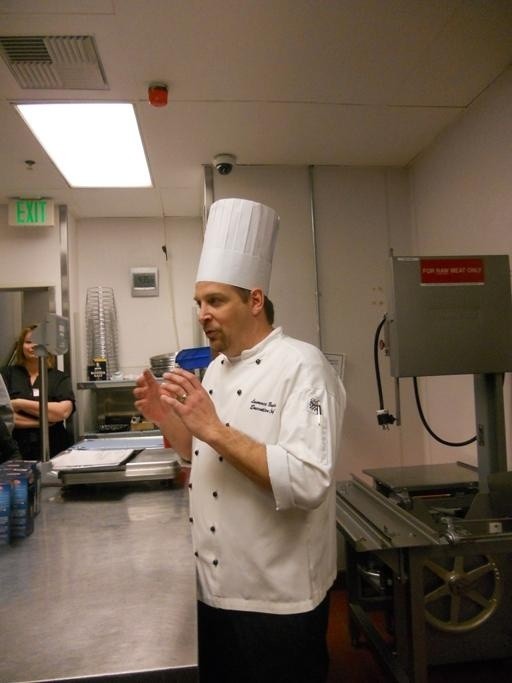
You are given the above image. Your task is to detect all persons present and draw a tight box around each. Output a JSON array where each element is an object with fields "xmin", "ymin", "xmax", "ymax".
[{"xmin": 2, "ymin": 325, "xmax": 76, "ymax": 460}]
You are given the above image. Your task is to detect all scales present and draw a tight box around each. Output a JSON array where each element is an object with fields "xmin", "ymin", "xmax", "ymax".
[{"xmin": 30, "ymin": 314, "xmax": 177, "ymax": 486}]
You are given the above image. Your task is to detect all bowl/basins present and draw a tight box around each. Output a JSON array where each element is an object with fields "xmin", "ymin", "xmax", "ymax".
[
  {"xmin": 150, "ymin": 366, "xmax": 180, "ymax": 378},
  {"xmin": 151, "ymin": 352, "xmax": 178, "ymax": 367}
]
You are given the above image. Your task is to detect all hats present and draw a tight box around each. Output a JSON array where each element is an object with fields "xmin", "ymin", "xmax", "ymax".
[{"xmin": 193, "ymin": 197, "xmax": 281, "ymax": 298}]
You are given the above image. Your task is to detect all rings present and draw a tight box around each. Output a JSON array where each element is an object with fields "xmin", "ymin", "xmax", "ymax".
[
  {"xmin": 132, "ymin": 197, "xmax": 346, "ymax": 682},
  {"xmin": 179, "ymin": 393, "xmax": 188, "ymax": 403}
]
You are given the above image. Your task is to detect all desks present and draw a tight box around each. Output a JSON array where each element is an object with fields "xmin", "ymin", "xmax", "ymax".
[{"xmin": 0, "ymin": 460, "xmax": 199, "ymax": 682}]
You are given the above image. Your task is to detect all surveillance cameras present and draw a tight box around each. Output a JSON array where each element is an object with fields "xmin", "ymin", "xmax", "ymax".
[{"xmin": 212, "ymin": 152, "xmax": 236, "ymax": 175}]
